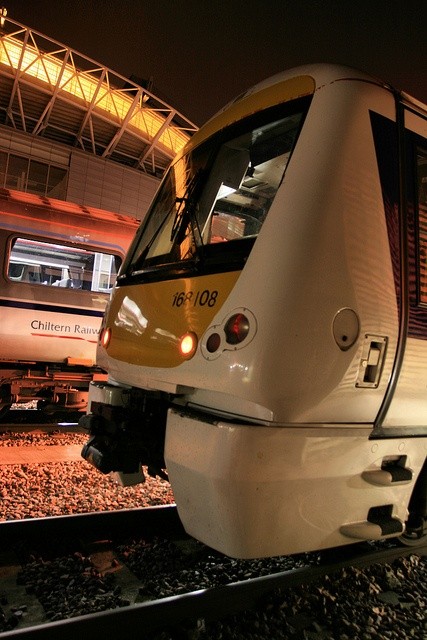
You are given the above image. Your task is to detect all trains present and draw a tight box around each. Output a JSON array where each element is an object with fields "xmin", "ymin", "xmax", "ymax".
[
  {"xmin": 0, "ymin": 187, "xmax": 142, "ymax": 418},
  {"xmin": 80, "ymin": 63, "xmax": 427, "ymax": 560}
]
